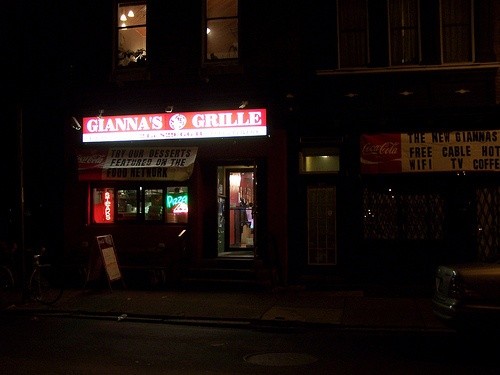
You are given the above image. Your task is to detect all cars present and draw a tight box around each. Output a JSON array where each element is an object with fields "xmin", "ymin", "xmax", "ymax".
[{"xmin": 425, "ymin": 260, "xmax": 500, "ymax": 327}]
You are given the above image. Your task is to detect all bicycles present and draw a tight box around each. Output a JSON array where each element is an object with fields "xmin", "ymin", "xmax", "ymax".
[{"xmin": 1, "ymin": 245, "xmax": 66, "ymax": 306}]
